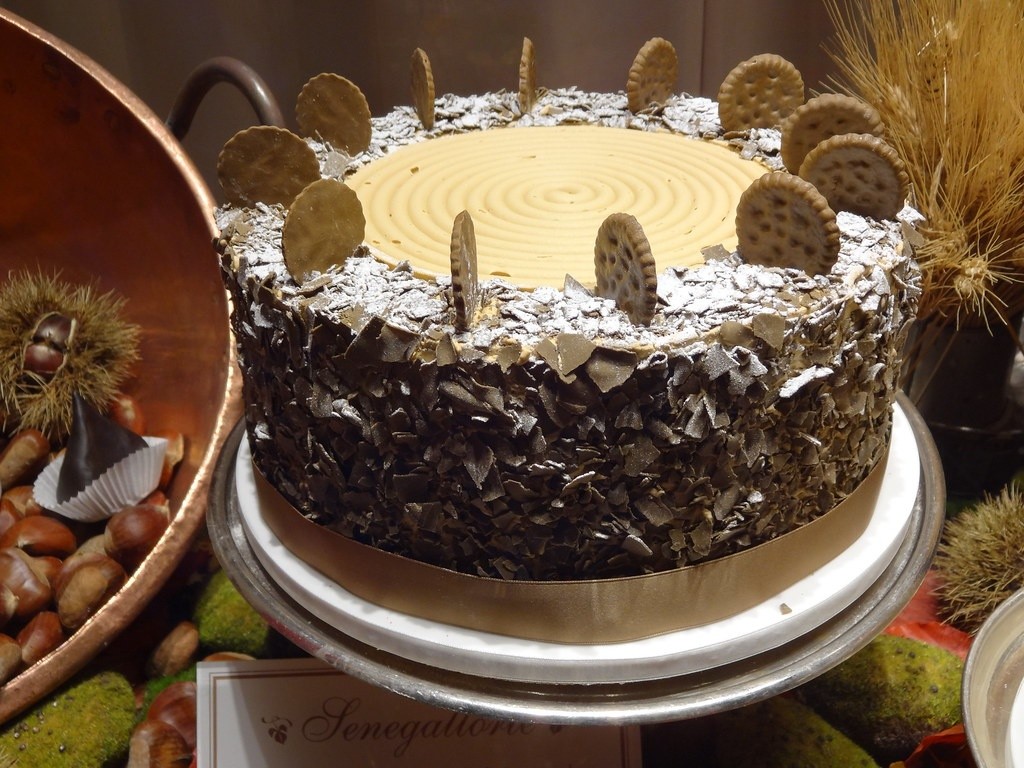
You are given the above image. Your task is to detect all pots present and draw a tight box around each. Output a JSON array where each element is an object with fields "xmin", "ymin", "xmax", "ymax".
[{"xmin": 0, "ymin": 0, "xmax": 285, "ymax": 718}]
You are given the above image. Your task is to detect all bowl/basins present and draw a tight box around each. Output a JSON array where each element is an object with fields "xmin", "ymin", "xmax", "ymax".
[{"xmin": 959, "ymin": 586, "xmax": 1024, "ymax": 768}]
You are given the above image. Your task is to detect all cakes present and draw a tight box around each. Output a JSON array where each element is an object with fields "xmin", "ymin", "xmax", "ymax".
[{"xmin": 211, "ymin": 38, "xmax": 925, "ymax": 645}]
